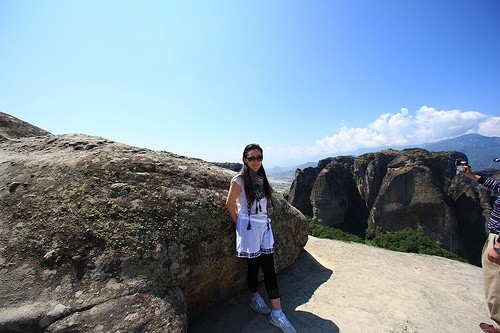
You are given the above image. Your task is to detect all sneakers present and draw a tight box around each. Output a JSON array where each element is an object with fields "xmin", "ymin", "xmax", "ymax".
[
  {"xmin": 270, "ymin": 311, "xmax": 296, "ymax": 333},
  {"xmin": 249, "ymin": 295, "xmax": 271, "ymax": 314}
]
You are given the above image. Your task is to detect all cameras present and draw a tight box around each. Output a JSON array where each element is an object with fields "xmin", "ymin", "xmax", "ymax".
[{"xmin": 456, "ymin": 166, "xmax": 466, "ymax": 174}]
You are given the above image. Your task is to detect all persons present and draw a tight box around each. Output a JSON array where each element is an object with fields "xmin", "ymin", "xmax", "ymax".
[
  {"xmin": 457, "ymin": 157, "xmax": 500, "ymax": 333},
  {"xmin": 226, "ymin": 144, "xmax": 296, "ymax": 333}
]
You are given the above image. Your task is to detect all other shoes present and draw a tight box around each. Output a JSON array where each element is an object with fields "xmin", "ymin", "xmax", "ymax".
[{"xmin": 480, "ymin": 323, "xmax": 500, "ymax": 333}]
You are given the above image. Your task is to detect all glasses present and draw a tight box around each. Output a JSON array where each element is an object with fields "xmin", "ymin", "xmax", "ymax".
[{"xmin": 246, "ymin": 156, "xmax": 263, "ymax": 162}]
